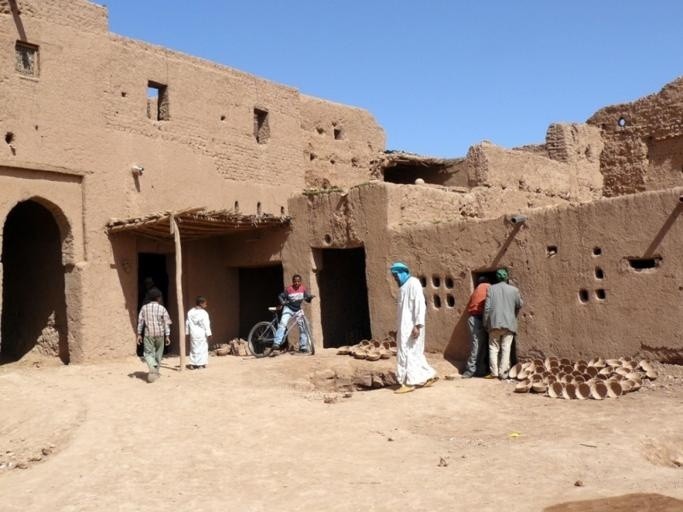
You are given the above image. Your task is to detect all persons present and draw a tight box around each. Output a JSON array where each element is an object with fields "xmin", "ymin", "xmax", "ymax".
[
  {"xmin": 272, "ymin": 274, "xmax": 316, "ymax": 352},
  {"xmin": 136, "ymin": 288, "xmax": 172, "ymax": 382},
  {"xmin": 460, "ymin": 276, "xmax": 492, "ymax": 379},
  {"xmin": 142, "ymin": 278, "xmax": 164, "ymax": 305},
  {"xmin": 390, "ymin": 262, "xmax": 439, "ymax": 393},
  {"xmin": 482, "ymin": 268, "xmax": 524, "ymax": 378},
  {"xmin": 185, "ymin": 295, "xmax": 213, "ymax": 370}
]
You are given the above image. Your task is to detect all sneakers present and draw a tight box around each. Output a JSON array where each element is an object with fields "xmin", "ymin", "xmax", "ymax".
[
  {"xmin": 483, "ymin": 373, "xmax": 496, "ymax": 378},
  {"xmin": 422, "ymin": 375, "xmax": 440, "ymax": 388},
  {"xmin": 392, "ymin": 385, "xmax": 415, "ymax": 394}
]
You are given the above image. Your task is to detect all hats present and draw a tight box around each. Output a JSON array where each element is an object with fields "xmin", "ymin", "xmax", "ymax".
[{"xmin": 497, "ymin": 269, "xmax": 509, "ymax": 281}]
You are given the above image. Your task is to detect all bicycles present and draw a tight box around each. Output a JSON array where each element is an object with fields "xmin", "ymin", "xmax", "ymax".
[{"xmin": 247, "ymin": 294, "xmax": 317, "ymax": 359}]
